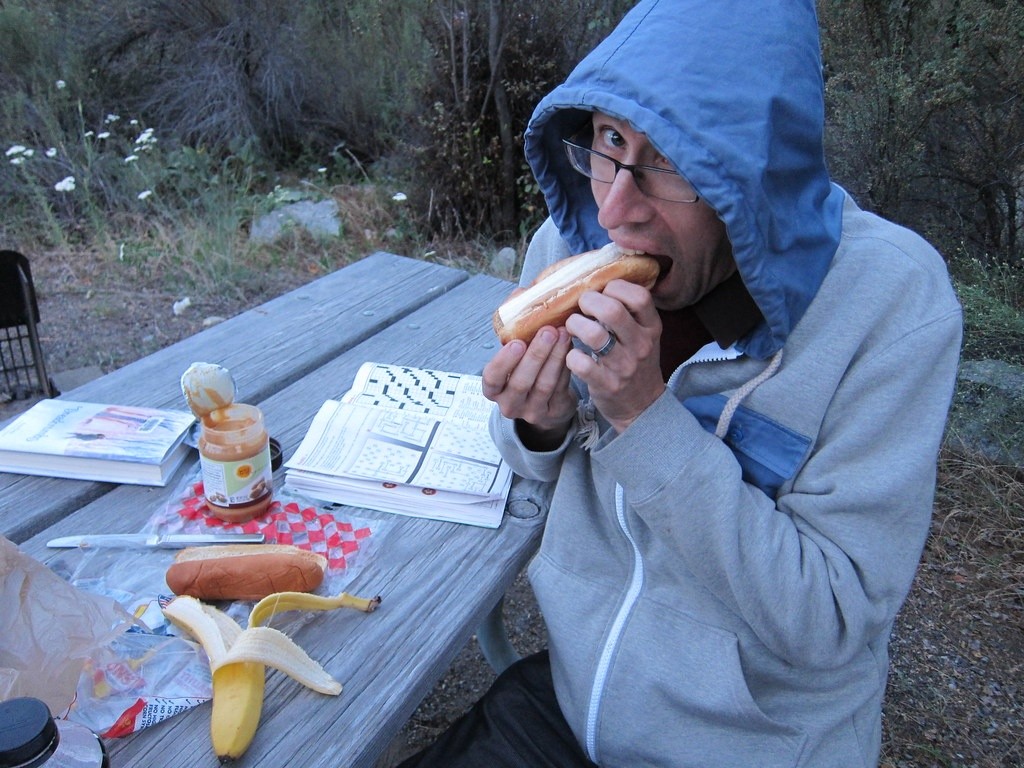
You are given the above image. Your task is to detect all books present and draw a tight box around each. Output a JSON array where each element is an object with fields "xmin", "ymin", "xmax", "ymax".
[
  {"xmin": 285, "ymin": 362, "xmax": 526, "ymax": 528},
  {"xmin": 0, "ymin": 396, "xmax": 203, "ymax": 488}
]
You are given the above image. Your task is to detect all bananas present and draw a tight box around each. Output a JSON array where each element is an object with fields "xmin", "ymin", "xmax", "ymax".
[{"xmin": 158, "ymin": 591, "xmax": 383, "ymax": 764}]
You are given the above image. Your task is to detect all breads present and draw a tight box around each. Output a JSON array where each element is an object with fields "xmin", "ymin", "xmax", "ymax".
[
  {"xmin": 491, "ymin": 241, "xmax": 661, "ymax": 346},
  {"xmin": 164, "ymin": 543, "xmax": 328, "ymax": 600}
]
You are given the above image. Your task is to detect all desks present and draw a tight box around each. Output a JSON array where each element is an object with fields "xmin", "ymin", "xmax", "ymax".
[{"xmin": 1, "ymin": 252, "xmax": 555, "ymax": 768}]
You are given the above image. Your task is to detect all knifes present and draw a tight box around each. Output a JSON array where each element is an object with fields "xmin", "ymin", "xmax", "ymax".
[{"xmin": 47, "ymin": 530, "xmax": 266, "ymax": 551}]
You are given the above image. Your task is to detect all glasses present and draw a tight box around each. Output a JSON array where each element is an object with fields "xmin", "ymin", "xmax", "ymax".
[{"xmin": 559, "ymin": 113, "xmax": 700, "ymax": 202}]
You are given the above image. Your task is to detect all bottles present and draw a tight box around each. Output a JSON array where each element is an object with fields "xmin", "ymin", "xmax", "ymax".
[
  {"xmin": 195, "ymin": 406, "xmax": 272, "ymax": 523},
  {"xmin": 0, "ymin": 696, "xmax": 110, "ymax": 768}
]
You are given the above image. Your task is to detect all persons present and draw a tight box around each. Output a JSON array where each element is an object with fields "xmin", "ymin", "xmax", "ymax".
[{"xmin": 390, "ymin": 1, "xmax": 967, "ymax": 768}]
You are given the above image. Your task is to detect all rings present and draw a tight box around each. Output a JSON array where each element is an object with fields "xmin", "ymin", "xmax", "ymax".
[{"xmin": 592, "ymin": 330, "xmax": 618, "ymax": 356}]
